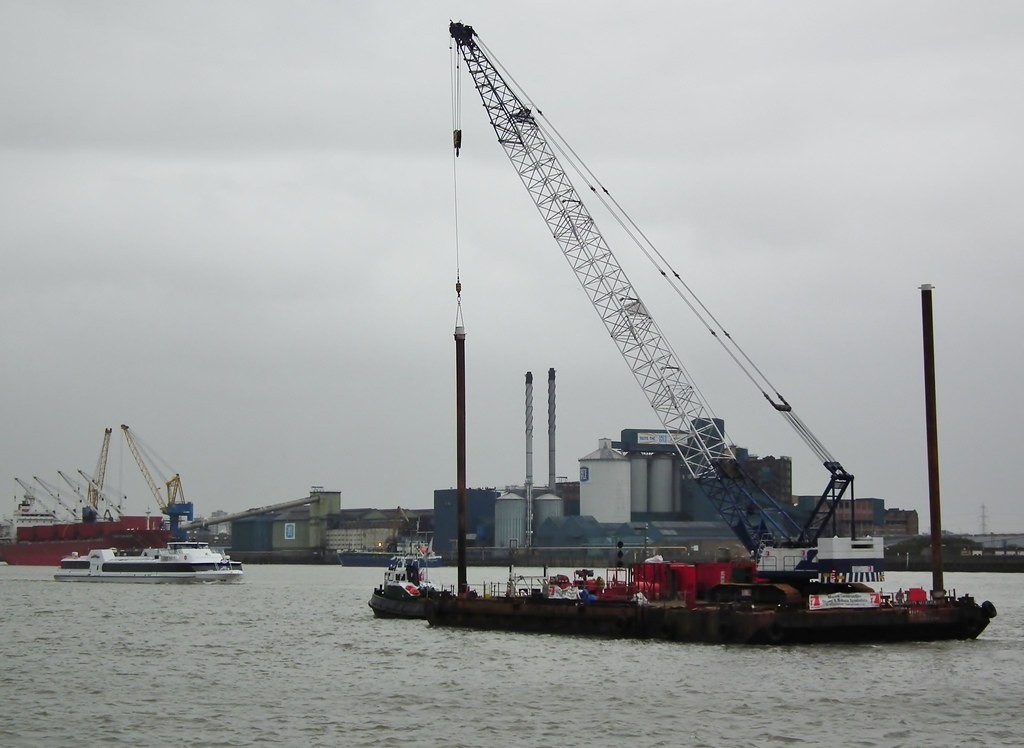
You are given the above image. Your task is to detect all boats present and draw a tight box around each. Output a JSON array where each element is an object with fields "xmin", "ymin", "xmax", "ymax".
[
  {"xmin": 54, "ymin": 538, "xmax": 245, "ymax": 586},
  {"xmin": 0, "ymin": 422, "xmax": 197, "ymax": 566},
  {"xmin": 368, "ymin": 553, "xmax": 443, "ymax": 620},
  {"xmin": 422, "ymin": 17, "xmax": 999, "ymax": 646},
  {"xmin": 337, "ymin": 506, "xmax": 443, "ymax": 569}
]
